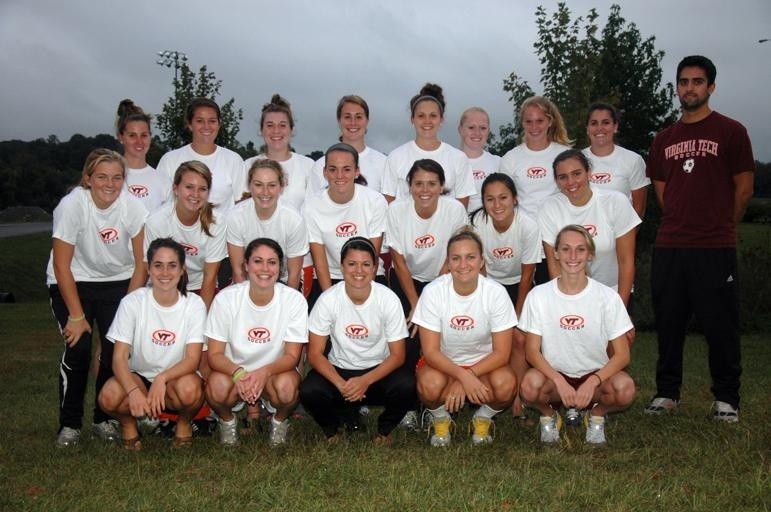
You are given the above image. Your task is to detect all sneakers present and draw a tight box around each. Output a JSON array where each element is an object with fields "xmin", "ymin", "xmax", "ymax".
[
  {"xmin": 513, "ymin": 407, "xmax": 606, "ymax": 445},
  {"xmin": 324, "ymin": 404, "xmax": 495, "ymax": 447},
  {"xmin": 57, "ymin": 411, "xmax": 290, "ymax": 448},
  {"xmin": 644, "ymin": 393, "xmax": 683, "ymax": 415},
  {"xmin": 709, "ymin": 398, "xmax": 741, "ymax": 425}
]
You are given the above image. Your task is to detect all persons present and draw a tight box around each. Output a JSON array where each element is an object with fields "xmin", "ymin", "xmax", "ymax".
[
  {"xmin": 645, "ymin": 55, "xmax": 757, "ymax": 425},
  {"xmin": 115, "ymin": 85, "xmax": 646, "ymax": 238},
  {"xmin": 201, "ymin": 239, "xmax": 311, "ymax": 448},
  {"xmin": 129, "ymin": 144, "xmax": 634, "ymax": 436},
  {"xmin": 100, "ymin": 236, "xmax": 208, "ymax": 453},
  {"xmin": 411, "ymin": 231, "xmax": 518, "ymax": 448},
  {"xmin": 46, "ymin": 147, "xmax": 148, "ymax": 447},
  {"xmin": 302, "ymin": 237, "xmax": 414, "ymax": 449},
  {"xmin": 521, "ymin": 223, "xmax": 635, "ymax": 443}
]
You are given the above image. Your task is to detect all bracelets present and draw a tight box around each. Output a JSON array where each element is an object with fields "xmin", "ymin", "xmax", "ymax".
[{"xmin": 67, "ymin": 314, "xmax": 86, "ymax": 322}]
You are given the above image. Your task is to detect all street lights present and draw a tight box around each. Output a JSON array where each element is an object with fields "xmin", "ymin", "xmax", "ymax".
[
  {"xmin": 154, "ymin": 48, "xmax": 189, "ymax": 143},
  {"xmin": 759, "ymin": 37, "xmax": 771, "ymax": 44}
]
